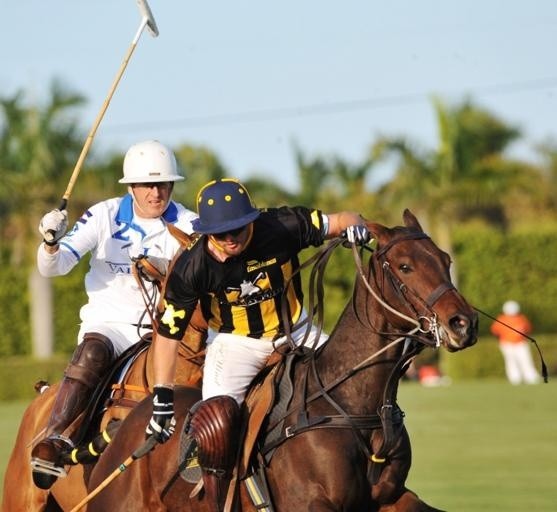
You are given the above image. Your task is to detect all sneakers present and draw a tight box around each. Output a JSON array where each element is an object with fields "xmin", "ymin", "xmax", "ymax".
[{"xmin": 29, "ymin": 439, "xmax": 63, "ymax": 491}]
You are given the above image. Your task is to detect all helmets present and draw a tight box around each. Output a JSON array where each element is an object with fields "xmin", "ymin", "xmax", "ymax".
[
  {"xmin": 118, "ymin": 140, "xmax": 185, "ymax": 184},
  {"xmin": 192, "ymin": 178, "xmax": 261, "ymax": 234}
]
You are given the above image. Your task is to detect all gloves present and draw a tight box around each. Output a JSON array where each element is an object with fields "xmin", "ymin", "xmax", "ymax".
[
  {"xmin": 132, "ymin": 254, "xmax": 169, "ymax": 283},
  {"xmin": 144, "ymin": 386, "xmax": 177, "ymax": 451},
  {"xmin": 39, "ymin": 209, "xmax": 69, "ymax": 245},
  {"xmin": 340, "ymin": 225, "xmax": 371, "ymax": 249}
]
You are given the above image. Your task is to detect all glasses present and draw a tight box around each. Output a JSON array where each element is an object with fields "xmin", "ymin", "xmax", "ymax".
[{"xmin": 212, "ymin": 227, "xmax": 245, "ymax": 239}]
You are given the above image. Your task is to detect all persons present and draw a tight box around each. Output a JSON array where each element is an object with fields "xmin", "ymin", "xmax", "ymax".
[
  {"xmin": 490, "ymin": 301, "xmax": 540, "ymax": 384},
  {"xmin": 144, "ymin": 178, "xmax": 375, "ymax": 485},
  {"xmin": 32, "ymin": 142, "xmax": 200, "ymax": 487},
  {"xmin": 401, "ymin": 360, "xmax": 439, "ymax": 385}
]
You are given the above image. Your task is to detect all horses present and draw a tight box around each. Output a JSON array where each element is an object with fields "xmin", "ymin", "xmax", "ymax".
[{"xmin": 0, "ymin": 207, "xmax": 480, "ymax": 512}]
